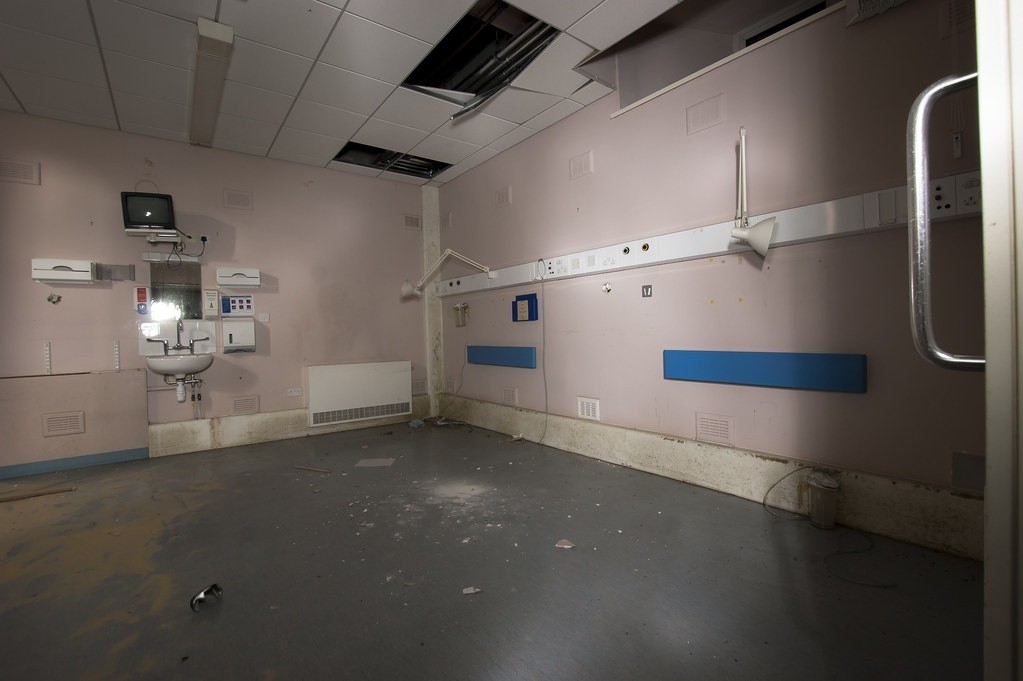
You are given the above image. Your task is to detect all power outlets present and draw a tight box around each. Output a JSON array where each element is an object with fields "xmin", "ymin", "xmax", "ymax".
[{"xmin": 955, "ymin": 170, "xmax": 982, "ymax": 216}]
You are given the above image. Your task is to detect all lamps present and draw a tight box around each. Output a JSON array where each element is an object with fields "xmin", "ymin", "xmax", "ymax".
[
  {"xmin": 398, "ymin": 248, "xmax": 492, "ymax": 299},
  {"xmin": 731, "ymin": 126, "xmax": 778, "ymax": 257}
]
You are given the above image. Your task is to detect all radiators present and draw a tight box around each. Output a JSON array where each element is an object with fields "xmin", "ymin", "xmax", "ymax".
[{"xmin": 303, "ymin": 360, "xmax": 413, "ymax": 429}]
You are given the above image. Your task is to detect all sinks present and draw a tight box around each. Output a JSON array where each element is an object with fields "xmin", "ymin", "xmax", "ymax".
[{"xmin": 144, "ymin": 352, "xmax": 213, "ymax": 376}]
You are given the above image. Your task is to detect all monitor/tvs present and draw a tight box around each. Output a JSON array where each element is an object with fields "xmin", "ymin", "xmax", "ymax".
[{"xmin": 121, "ymin": 192, "xmax": 174, "ymax": 230}]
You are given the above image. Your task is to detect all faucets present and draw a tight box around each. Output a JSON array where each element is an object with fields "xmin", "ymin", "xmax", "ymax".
[{"xmin": 175, "ymin": 319, "xmax": 185, "ymax": 350}]
